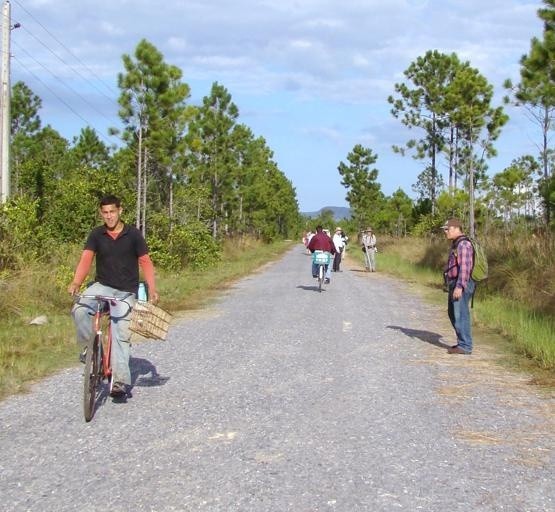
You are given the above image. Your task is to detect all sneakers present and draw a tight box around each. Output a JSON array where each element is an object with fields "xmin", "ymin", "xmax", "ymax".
[
  {"xmin": 448, "ymin": 346, "xmax": 473, "ymax": 355},
  {"xmin": 111, "ymin": 382, "xmax": 128, "ymax": 398}
]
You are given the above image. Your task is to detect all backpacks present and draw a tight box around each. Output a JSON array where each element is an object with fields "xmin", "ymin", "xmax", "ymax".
[{"xmin": 469, "ymin": 237, "xmax": 489, "ymax": 282}]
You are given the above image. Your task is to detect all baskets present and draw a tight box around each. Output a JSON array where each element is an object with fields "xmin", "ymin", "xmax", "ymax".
[{"xmin": 127, "ymin": 298, "xmax": 174, "ymax": 344}]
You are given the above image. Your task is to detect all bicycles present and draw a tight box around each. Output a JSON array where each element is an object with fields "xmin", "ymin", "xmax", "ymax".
[
  {"xmin": 312, "ymin": 250, "xmax": 333, "ymax": 292},
  {"xmin": 71, "ymin": 289, "xmax": 133, "ymax": 421}
]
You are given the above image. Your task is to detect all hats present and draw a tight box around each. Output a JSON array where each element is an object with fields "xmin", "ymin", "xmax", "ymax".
[
  {"xmin": 366, "ymin": 226, "xmax": 373, "ymax": 231},
  {"xmin": 440, "ymin": 219, "xmax": 462, "ymax": 229}
]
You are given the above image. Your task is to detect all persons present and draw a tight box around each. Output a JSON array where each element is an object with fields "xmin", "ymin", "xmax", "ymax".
[
  {"xmin": 67, "ymin": 196, "xmax": 158, "ymax": 398},
  {"xmin": 361, "ymin": 227, "xmax": 377, "ymax": 272},
  {"xmin": 442, "ymin": 218, "xmax": 477, "ymax": 354},
  {"xmin": 302, "ymin": 225, "xmax": 348, "ymax": 284}
]
